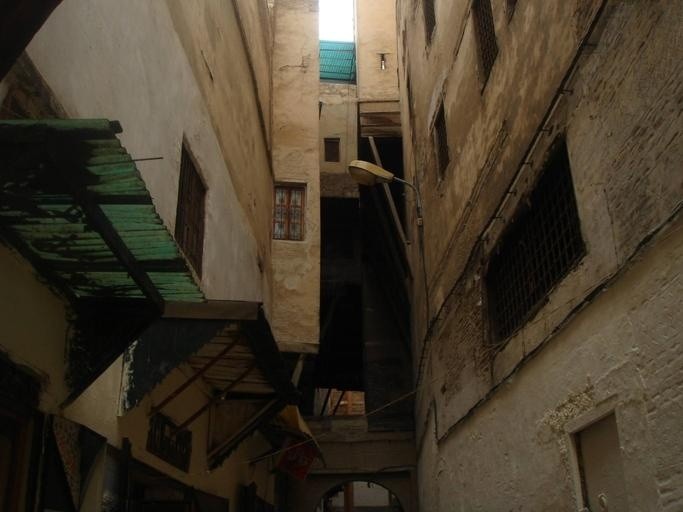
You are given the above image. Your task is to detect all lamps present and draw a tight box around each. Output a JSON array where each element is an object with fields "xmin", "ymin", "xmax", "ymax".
[{"xmin": 348, "ymin": 159, "xmax": 423, "ymax": 227}]
